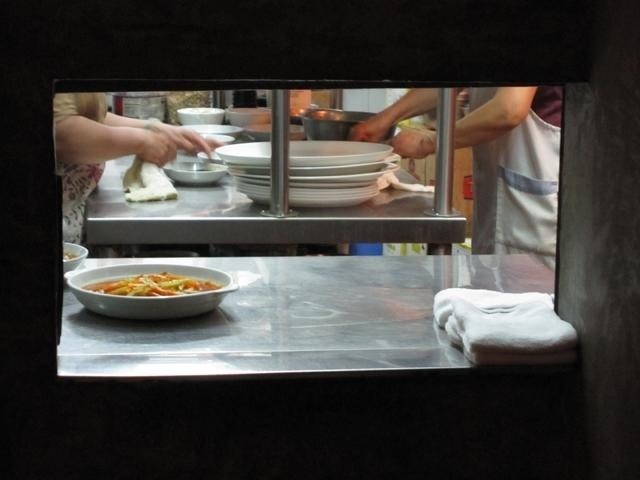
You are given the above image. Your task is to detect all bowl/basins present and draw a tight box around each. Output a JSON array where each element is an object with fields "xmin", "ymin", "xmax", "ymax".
[
  {"xmin": 303, "ymin": 108, "xmax": 396, "ymax": 141},
  {"xmin": 60, "ymin": 238, "xmax": 88, "ymax": 277},
  {"xmin": 177, "ymin": 106, "xmax": 273, "ymax": 125}
]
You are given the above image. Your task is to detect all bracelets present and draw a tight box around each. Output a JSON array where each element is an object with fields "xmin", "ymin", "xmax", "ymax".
[{"xmin": 144, "ymin": 114, "xmax": 160, "ymax": 130}]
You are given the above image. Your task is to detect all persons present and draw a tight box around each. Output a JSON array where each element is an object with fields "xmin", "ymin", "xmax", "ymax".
[
  {"xmin": 54, "ymin": 92, "xmax": 225, "ymax": 254},
  {"xmin": 351, "ymin": 85, "xmax": 564, "ymax": 272}
]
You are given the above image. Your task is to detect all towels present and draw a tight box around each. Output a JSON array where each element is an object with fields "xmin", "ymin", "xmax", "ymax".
[
  {"xmin": 123, "ymin": 156, "xmax": 177, "ymax": 201},
  {"xmin": 433, "ymin": 286, "xmax": 579, "ymax": 369}
]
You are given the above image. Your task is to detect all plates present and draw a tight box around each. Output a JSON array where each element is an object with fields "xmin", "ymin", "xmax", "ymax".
[
  {"xmin": 63, "ymin": 262, "xmax": 241, "ymax": 320},
  {"xmin": 162, "ymin": 125, "xmax": 401, "ymax": 207}
]
